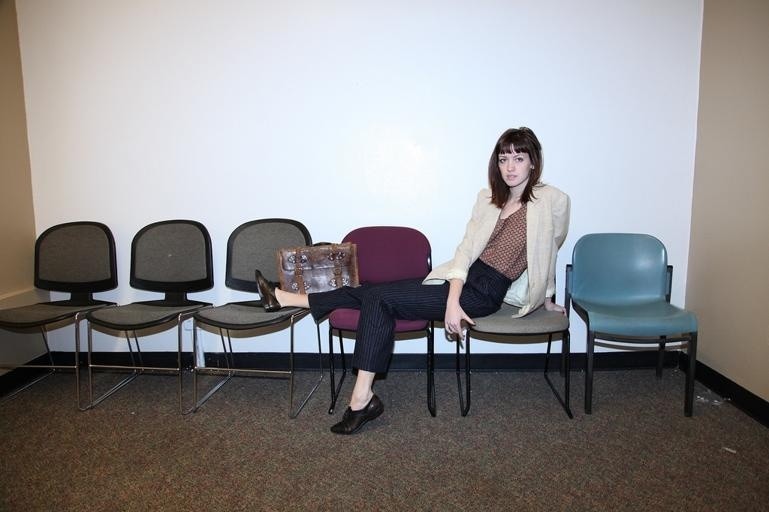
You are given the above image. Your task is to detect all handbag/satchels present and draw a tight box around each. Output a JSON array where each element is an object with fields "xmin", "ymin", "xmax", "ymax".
[{"xmin": 276, "ymin": 241, "xmax": 360, "ymax": 295}]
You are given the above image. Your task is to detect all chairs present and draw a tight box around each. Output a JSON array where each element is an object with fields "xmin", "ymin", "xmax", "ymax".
[
  {"xmin": 1, "ymin": 221, "xmax": 138, "ymax": 411},
  {"xmin": 194, "ymin": 223, "xmax": 327, "ymax": 420},
  {"xmin": 84, "ymin": 219, "xmax": 233, "ymax": 415},
  {"xmin": 456, "ymin": 274, "xmax": 577, "ymax": 421},
  {"xmin": 319, "ymin": 227, "xmax": 440, "ymax": 417},
  {"xmin": 566, "ymin": 232, "xmax": 699, "ymax": 414}
]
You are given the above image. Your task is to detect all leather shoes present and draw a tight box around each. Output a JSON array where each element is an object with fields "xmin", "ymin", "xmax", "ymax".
[
  {"xmin": 330, "ymin": 393, "xmax": 384, "ymax": 435},
  {"xmin": 255, "ymin": 268, "xmax": 281, "ymax": 313}
]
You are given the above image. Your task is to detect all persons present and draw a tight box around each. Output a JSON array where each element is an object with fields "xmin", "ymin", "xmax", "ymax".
[{"xmin": 254, "ymin": 125, "xmax": 571, "ymax": 436}]
